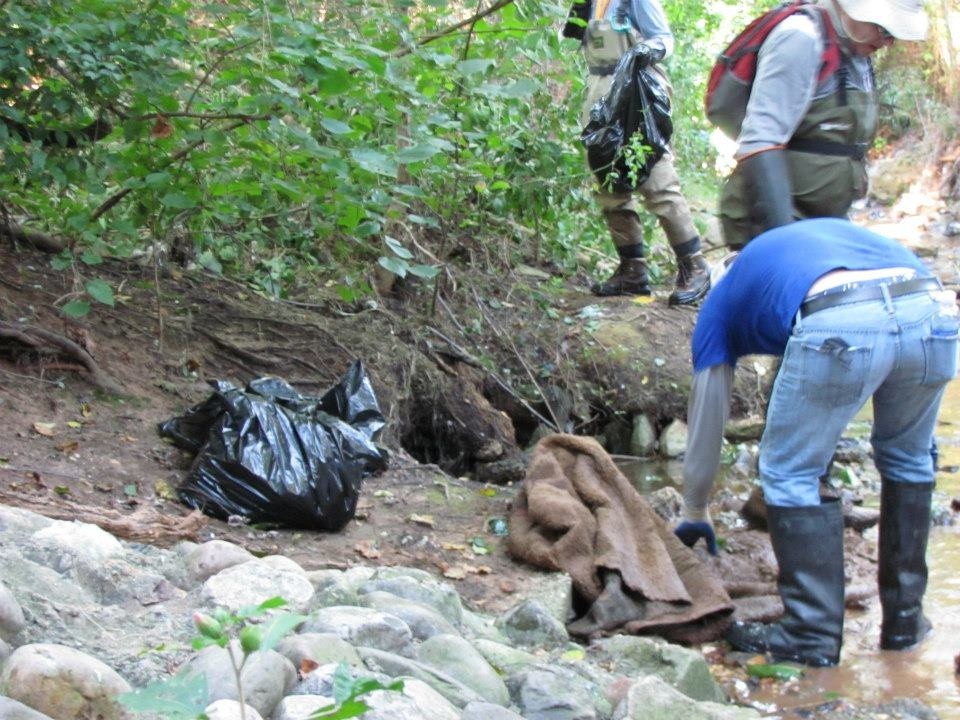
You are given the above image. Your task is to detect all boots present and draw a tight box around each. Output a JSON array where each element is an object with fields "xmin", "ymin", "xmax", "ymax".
[
  {"xmin": 878, "ymin": 478, "xmax": 936, "ymax": 654},
  {"xmin": 720, "ymin": 495, "xmax": 846, "ymax": 667}
]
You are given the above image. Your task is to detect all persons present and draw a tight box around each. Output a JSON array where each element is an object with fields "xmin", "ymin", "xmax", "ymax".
[
  {"xmin": 675, "ymin": 218, "xmax": 959, "ymax": 668},
  {"xmin": 558, "ymin": 1, "xmax": 712, "ymax": 307},
  {"xmin": 719, "ymin": 1, "xmax": 930, "ymax": 531}
]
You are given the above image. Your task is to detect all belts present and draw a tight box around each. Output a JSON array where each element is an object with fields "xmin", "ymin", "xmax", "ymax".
[{"xmin": 794, "ymin": 276, "xmax": 943, "ymax": 323}]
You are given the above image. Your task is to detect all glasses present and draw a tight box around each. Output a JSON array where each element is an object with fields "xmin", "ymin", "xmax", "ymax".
[{"xmin": 874, "ymin": 25, "xmax": 891, "ymax": 39}]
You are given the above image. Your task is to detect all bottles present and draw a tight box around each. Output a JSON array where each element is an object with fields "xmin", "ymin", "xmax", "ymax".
[{"xmin": 937, "ymin": 289, "xmax": 959, "ymax": 318}]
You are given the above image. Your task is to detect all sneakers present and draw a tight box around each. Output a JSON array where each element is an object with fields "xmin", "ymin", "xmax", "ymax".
[
  {"xmin": 591, "ymin": 259, "xmax": 652, "ymax": 296},
  {"xmin": 667, "ymin": 250, "xmax": 713, "ymax": 303}
]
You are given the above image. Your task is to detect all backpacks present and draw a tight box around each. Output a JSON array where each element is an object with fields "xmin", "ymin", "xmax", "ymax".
[{"xmin": 701, "ymin": 0, "xmax": 842, "ymax": 143}]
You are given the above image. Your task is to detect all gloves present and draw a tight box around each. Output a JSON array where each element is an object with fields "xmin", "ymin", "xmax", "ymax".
[{"xmin": 674, "ymin": 505, "xmax": 717, "ymax": 555}]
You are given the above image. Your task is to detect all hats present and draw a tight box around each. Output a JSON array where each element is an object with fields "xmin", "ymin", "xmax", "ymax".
[{"xmin": 834, "ymin": 0, "xmax": 932, "ymax": 42}]
